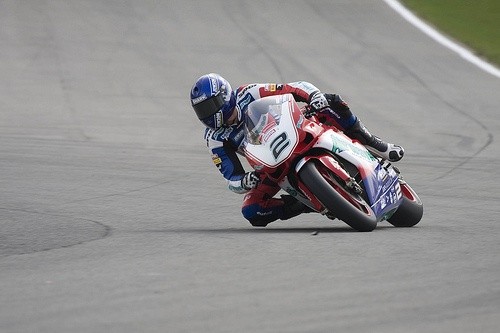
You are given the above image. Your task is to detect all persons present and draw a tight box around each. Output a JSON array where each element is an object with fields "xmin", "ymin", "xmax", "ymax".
[{"xmin": 189, "ymin": 73, "xmax": 405, "ymax": 227}]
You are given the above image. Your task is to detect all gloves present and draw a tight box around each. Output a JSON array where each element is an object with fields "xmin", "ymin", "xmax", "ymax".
[
  {"xmin": 308, "ymin": 91, "xmax": 328, "ymax": 112},
  {"xmin": 241, "ymin": 171, "xmax": 260, "ymax": 190}
]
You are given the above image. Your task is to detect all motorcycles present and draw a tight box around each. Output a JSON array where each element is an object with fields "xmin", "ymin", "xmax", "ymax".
[{"xmin": 242, "ymin": 94, "xmax": 423, "ymax": 232}]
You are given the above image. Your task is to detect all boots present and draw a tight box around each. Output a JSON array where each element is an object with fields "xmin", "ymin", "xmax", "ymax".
[
  {"xmin": 344, "ymin": 116, "xmax": 404, "ymax": 162},
  {"xmin": 279, "ymin": 194, "xmax": 336, "ymax": 220}
]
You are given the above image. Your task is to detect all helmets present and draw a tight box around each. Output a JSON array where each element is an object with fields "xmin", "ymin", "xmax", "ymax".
[{"xmin": 190, "ymin": 73, "xmax": 236, "ymax": 130}]
